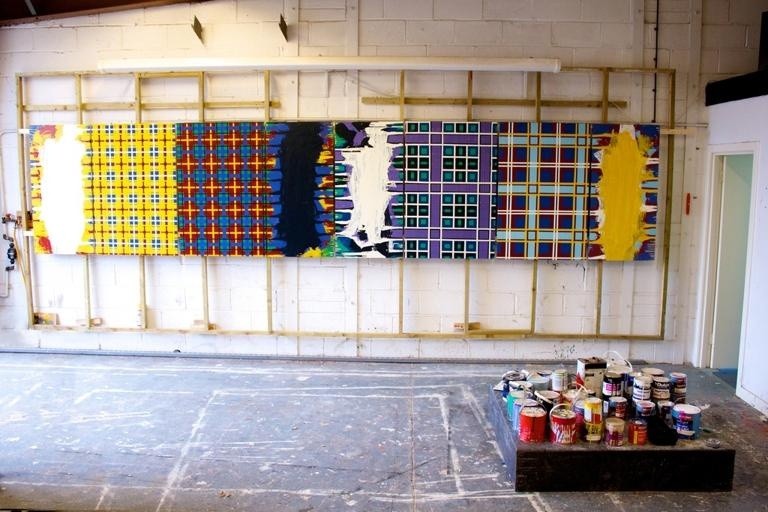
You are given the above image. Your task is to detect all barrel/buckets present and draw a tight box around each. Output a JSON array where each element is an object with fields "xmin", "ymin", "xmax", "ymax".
[
  {"xmin": 671, "ymin": 397, "xmax": 701, "ymax": 440},
  {"xmin": 602, "ymin": 351, "xmax": 633, "ymax": 393},
  {"xmin": 503, "ymin": 370, "xmax": 587, "ymax": 445}
]
